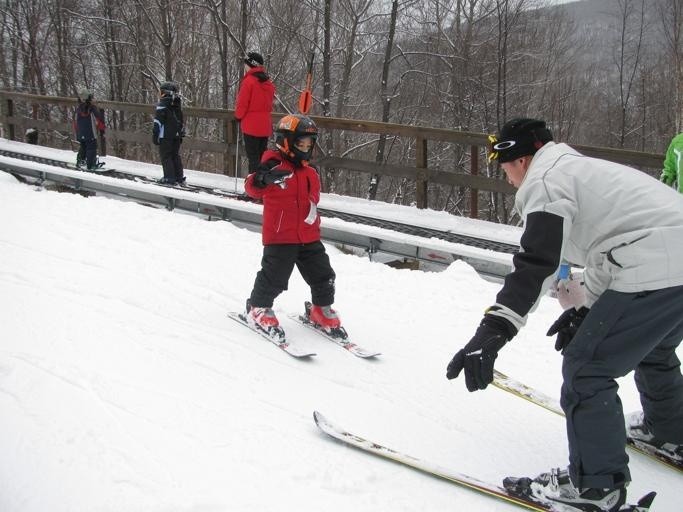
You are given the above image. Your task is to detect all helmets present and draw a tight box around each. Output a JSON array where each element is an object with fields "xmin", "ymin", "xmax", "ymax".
[{"xmin": 272, "ymin": 114, "xmax": 318, "ymax": 162}]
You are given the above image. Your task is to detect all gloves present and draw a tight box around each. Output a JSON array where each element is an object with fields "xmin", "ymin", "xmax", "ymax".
[
  {"xmin": 254, "ymin": 168, "xmax": 293, "ymax": 190},
  {"xmin": 445, "ymin": 312, "xmax": 521, "ymax": 392},
  {"xmin": 544, "ymin": 304, "xmax": 592, "ymax": 359}
]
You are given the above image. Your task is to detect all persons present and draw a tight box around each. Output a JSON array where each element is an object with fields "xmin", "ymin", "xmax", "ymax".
[
  {"xmin": 441, "ymin": 115, "xmax": 682, "ymax": 512},
  {"xmin": 231, "ymin": 51, "xmax": 276, "ymax": 196},
  {"xmin": 241, "ymin": 112, "xmax": 344, "ymax": 344},
  {"xmin": 659, "ymin": 131, "xmax": 683, "ymax": 195},
  {"xmin": 71, "ymin": 88, "xmax": 105, "ymax": 170},
  {"xmin": 151, "ymin": 81, "xmax": 184, "ymax": 183}
]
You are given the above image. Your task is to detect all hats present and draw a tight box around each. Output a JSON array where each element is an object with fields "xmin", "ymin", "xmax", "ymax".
[
  {"xmin": 243, "ymin": 51, "xmax": 263, "ymax": 69},
  {"xmin": 77, "ymin": 87, "xmax": 95, "ymax": 98},
  {"xmin": 489, "ymin": 117, "xmax": 555, "ymax": 163},
  {"xmin": 160, "ymin": 81, "xmax": 177, "ymax": 94}
]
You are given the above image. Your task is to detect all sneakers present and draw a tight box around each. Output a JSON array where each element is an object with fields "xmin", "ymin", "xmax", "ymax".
[
  {"xmin": 533, "ymin": 467, "xmax": 629, "ymax": 511},
  {"xmin": 306, "ymin": 302, "xmax": 343, "ymax": 332},
  {"xmin": 158, "ymin": 175, "xmax": 187, "ymax": 185},
  {"xmin": 75, "ymin": 159, "xmax": 106, "ymax": 170},
  {"xmin": 624, "ymin": 409, "xmax": 683, "ymax": 461},
  {"xmin": 243, "ymin": 303, "xmax": 281, "ymax": 334}
]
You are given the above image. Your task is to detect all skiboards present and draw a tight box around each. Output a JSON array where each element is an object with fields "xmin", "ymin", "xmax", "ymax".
[
  {"xmin": 313, "ymin": 369, "xmax": 683, "ymax": 512},
  {"xmin": 66, "ymin": 162, "xmax": 116, "ymax": 173},
  {"xmin": 134, "ymin": 175, "xmax": 201, "ymax": 191},
  {"xmin": 228, "ymin": 310, "xmax": 381, "ymax": 358},
  {"xmin": 212, "ymin": 188, "xmax": 262, "ymax": 204}
]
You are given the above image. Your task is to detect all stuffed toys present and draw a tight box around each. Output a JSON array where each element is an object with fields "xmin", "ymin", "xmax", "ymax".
[{"xmin": 552, "ymin": 270, "xmax": 591, "ymax": 309}]
